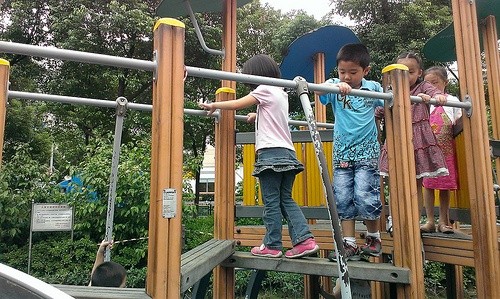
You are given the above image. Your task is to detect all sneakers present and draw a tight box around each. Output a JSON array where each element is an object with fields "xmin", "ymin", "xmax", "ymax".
[
  {"xmin": 328, "ymin": 239, "xmax": 362, "ymax": 263},
  {"xmin": 284, "ymin": 239, "xmax": 319, "ymax": 258},
  {"xmin": 359, "ymin": 235, "xmax": 383, "ymax": 256},
  {"xmin": 250, "ymin": 245, "xmax": 283, "ymax": 259}
]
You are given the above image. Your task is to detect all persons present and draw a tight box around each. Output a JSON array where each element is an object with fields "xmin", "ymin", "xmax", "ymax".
[
  {"xmin": 198, "ymin": 55, "xmax": 320, "ymax": 259},
  {"xmin": 88, "ymin": 239, "xmax": 126, "ymax": 288},
  {"xmin": 315, "ymin": 42, "xmax": 384, "ymax": 262},
  {"xmin": 420, "ymin": 66, "xmax": 463, "ymax": 233},
  {"xmin": 375, "ymin": 52, "xmax": 449, "ymax": 237}
]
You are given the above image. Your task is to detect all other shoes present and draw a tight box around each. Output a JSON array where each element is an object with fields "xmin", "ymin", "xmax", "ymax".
[
  {"xmin": 386, "ymin": 226, "xmax": 393, "ymax": 239},
  {"xmin": 420, "ymin": 222, "xmax": 437, "ymax": 234},
  {"xmin": 437, "ymin": 223, "xmax": 454, "ymax": 234}
]
